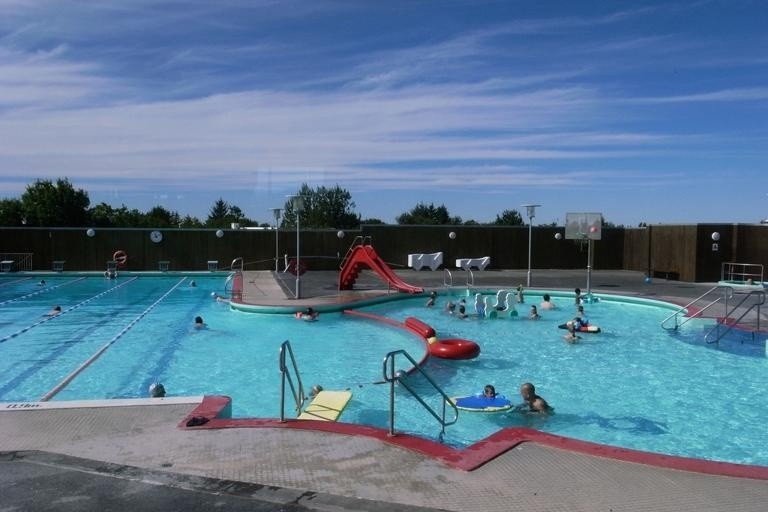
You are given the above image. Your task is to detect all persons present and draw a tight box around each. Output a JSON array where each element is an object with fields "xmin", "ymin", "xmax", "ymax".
[
  {"xmin": 513, "ymin": 381, "xmax": 553, "ymax": 419},
  {"xmin": 425, "ymin": 283, "xmax": 589, "ymax": 343},
  {"xmin": 189, "ymin": 280, "xmax": 197, "ymax": 287},
  {"xmin": 310, "ymin": 384, "xmax": 323, "ymax": 397},
  {"xmin": 149, "ymin": 380, "xmax": 167, "ymax": 399},
  {"xmin": 192, "ymin": 316, "xmax": 207, "ymax": 330},
  {"xmin": 43, "ymin": 305, "xmax": 62, "ymax": 319},
  {"xmin": 483, "ymin": 384, "xmax": 496, "ymax": 399},
  {"xmin": 211, "ymin": 291, "xmax": 229, "ymax": 303},
  {"xmin": 295, "ymin": 305, "xmax": 320, "ymax": 321},
  {"xmin": 38, "ymin": 278, "xmax": 47, "ymax": 286}
]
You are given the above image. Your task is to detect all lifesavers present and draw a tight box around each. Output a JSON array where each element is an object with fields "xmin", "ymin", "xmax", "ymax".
[
  {"xmin": 430, "ymin": 339, "xmax": 479, "ymax": 359},
  {"xmin": 288, "ymin": 259, "xmax": 307, "ymax": 275},
  {"xmin": 113, "ymin": 250, "xmax": 128, "ymax": 266}
]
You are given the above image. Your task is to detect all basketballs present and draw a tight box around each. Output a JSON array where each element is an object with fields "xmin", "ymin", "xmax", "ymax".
[
  {"xmin": 591, "ymin": 226, "xmax": 597, "ymax": 232},
  {"xmin": 644, "ymin": 277, "xmax": 652, "ymax": 284}
]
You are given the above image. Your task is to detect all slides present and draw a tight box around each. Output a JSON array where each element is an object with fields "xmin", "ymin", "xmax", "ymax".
[{"xmin": 358, "ymin": 245, "xmax": 425, "ymax": 293}]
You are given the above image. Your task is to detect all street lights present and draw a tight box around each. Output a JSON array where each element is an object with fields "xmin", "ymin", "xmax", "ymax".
[
  {"xmin": 269, "ymin": 206, "xmax": 286, "ymax": 275},
  {"xmin": 287, "ymin": 192, "xmax": 309, "ymax": 301},
  {"xmin": 521, "ymin": 203, "xmax": 541, "ymax": 288}
]
